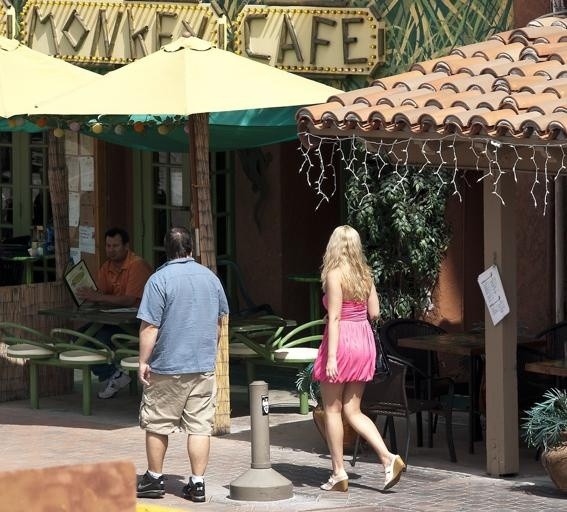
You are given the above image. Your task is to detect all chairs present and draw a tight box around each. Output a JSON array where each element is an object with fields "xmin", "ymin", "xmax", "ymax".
[
  {"xmin": 228, "ymin": 314, "xmax": 326, "ymax": 416},
  {"xmin": 480, "ymin": 345, "xmax": 559, "ymax": 460},
  {"xmin": 535, "ymin": 320, "xmax": 567, "ymax": 354},
  {"xmin": 351, "ymin": 353, "xmax": 457, "ymax": 472},
  {"xmin": 0, "ymin": 322, "xmax": 140, "ymax": 422},
  {"xmin": 380, "ymin": 318, "xmax": 474, "ymax": 454}
]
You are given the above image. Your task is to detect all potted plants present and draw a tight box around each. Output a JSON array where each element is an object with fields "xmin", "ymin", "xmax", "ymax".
[
  {"xmin": 520, "ymin": 388, "xmax": 567, "ymax": 493},
  {"xmin": 294, "ymin": 364, "xmax": 354, "ymax": 451}
]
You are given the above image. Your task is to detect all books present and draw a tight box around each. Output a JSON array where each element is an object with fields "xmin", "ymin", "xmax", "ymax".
[{"xmin": 62, "ymin": 256, "xmax": 99, "ymax": 308}]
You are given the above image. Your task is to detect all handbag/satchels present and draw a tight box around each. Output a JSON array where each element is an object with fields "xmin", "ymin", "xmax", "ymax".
[{"xmin": 364, "ymin": 319, "xmax": 392, "ymax": 385}]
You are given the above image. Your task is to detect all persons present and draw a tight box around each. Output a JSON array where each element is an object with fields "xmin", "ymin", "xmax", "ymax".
[
  {"xmin": 312, "ymin": 224, "xmax": 409, "ymax": 493},
  {"xmin": 68, "ymin": 229, "xmax": 157, "ymax": 400},
  {"xmin": 134, "ymin": 226, "xmax": 232, "ymax": 504}
]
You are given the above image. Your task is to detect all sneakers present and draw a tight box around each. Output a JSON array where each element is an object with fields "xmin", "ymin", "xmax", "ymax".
[
  {"xmin": 98, "ymin": 371, "xmax": 132, "ymax": 399},
  {"xmin": 181, "ymin": 477, "xmax": 205, "ymax": 502},
  {"xmin": 136, "ymin": 470, "xmax": 168, "ymax": 498}
]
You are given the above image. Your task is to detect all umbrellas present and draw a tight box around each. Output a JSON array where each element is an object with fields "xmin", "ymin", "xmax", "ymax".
[
  {"xmin": 2, "ymin": 31, "xmax": 103, "ymax": 136},
  {"xmin": 26, "ymin": 31, "xmax": 345, "ymax": 266}
]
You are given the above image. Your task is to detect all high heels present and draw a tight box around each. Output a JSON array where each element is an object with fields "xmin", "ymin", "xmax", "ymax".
[
  {"xmin": 383, "ymin": 455, "xmax": 406, "ymax": 491},
  {"xmin": 320, "ymin": 476, "xmax": 349, "ymax": 492}
]
